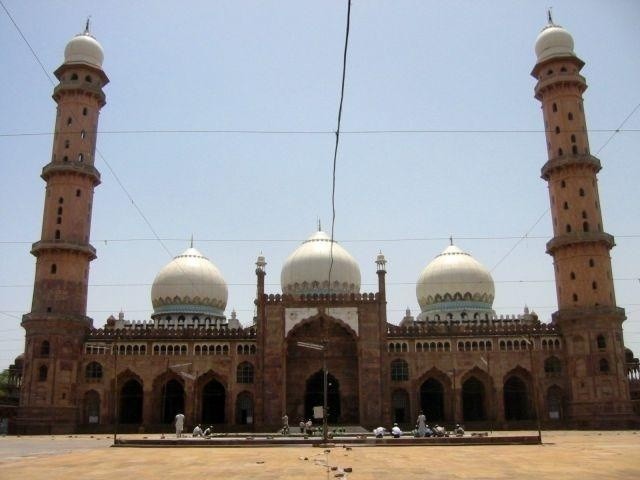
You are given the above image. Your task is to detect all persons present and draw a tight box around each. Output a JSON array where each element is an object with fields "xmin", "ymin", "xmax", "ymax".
[
  {"xmin": 281, "ymin": 413, "xmax": 289, "ymax": 436},
  {"xmin": 193, "ymin": 424, "xmax": 203, "ymax": 436},
  {"xmin": 299, "ymin": 419, "xmax": 313, "ymax": 435},
  {"xmin": 174, "ymin": 410, "xmax": 186, "ymax": 437},
  {"xmin": 373, "ymin": 410, "xmax": 464, "ymax": 438},
  {"xmin": 204, "ymin": 426, "xmax": 214, "ymax": 436}
]
